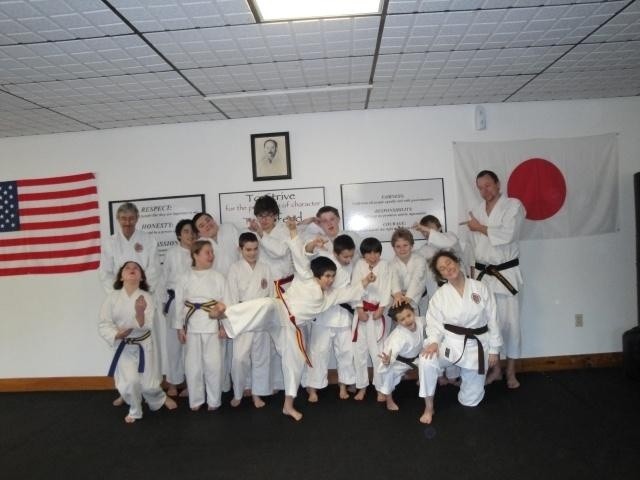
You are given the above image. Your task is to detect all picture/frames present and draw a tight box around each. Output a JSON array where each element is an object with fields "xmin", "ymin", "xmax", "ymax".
[
  {"xmin": 219, "ymin": 187, "xmax": 326, "ymax": 234},
  {"xmin": 108, "ymin": 194, "xmax": 205, "ymax": 265},
  {"xmin": 339, "ymin": 179, "xmax": 447, "ymax": 243},
  {"xmin": 251, "ymin": 131, "xmax": 292, "ymax": 180}
]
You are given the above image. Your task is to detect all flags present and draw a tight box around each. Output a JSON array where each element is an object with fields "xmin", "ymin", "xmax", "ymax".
[
  {"xmin": 452, "ymin": 132, "xmax": 622, "ymax": 242},
  {"xmin": 0, "ymin": 173, "xmax": 101, "ymax": 276}
]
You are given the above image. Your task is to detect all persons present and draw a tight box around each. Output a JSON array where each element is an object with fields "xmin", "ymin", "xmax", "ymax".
[
  {"xmin": 218, "ymin": 218, "xmax": 377, "ymax": 423},
  {"xmin": 301, "ymin": 234, "xmax": 356, "ymax": 402},
  {"xmin": 163, "ymin": 220, "xmax": 198, "ymax": 397},
  {"xmin": 458, "ymin": 171, "xmax": 527, "ymax": 389},
  {"xmin": 294, "ymin": 206, "xmax": 358, "ymax": 394},
  {"xmin": 243, "ymin": 196, "xmax": 296, "ymax": 397},
  {"xmin": 408, "ymin": 216, "xmax": 463, "ymax": 385},
  {"xmin": 387, "ymin": 225, "xmax": 428, "ymax": 387},
  {"xmin": 418, "ymin": 249, "xmax": 502, "ymax": 425},
  {"xmin": 352, "ymin": 237, "xmax": 390, "ymax": 403},
  {"xmin": 172, "ymin": 240, "xmax": 229, "ymax": 410},
  {"xmin": 195, "ymin": 214, "xmax": 241, "ymax": 392},
  {"xmin": 257, "ymin": 139, "xmax": 285, "ymax": 177},
  {"xmin": 97, "ymin": 261, "xmax": 177, "ymax": 423},
  {"xmin": 99, "ymin": 202, "xmax": 158, "ymax": 405},
  {"xmin": 225, "ymin": 233, "xmax": 273, "ymax": 409},
  {"xmin": 377, "ymin": 303, "xmax": 461, "ymax": 412}
]
